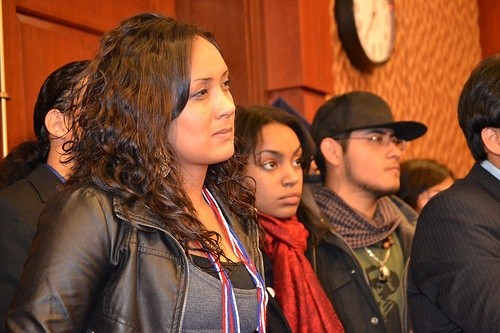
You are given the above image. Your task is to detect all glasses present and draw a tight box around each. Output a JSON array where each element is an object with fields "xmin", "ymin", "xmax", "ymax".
[{"xmin": 348, "ymin": 135, "xmax": 406, "ymax": 151}]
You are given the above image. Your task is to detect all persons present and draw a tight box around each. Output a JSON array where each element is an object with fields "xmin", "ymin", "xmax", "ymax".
[
  {"xmin": 7, "ymin": 16, "xmax": 292, "ymax": 333},
  {"xmin": 234, "ymin": 107, "xmax": 387, "ymax": 333},
  {"xmin": 410, "ymin": 51, "xmax": 500, "ymax": 333},
  {"xmin": 395, "ymin": 158, "xmax": 456, "ymax": 214},
  {"xmin": 0, "ymin": 57, "xmax": 100, "ymax": 333},
  {"xmin": 307, "ymin": 90, "xmax": 428, "ymax": 333}
]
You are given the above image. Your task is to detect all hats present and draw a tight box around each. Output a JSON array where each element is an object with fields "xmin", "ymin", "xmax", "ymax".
[{"xmin": 311, "ymin": 90, "xmax": 428, "ymax": 142}]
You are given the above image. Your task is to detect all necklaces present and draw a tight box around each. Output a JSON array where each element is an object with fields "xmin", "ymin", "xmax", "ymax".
[
  {"xmin": 364, "ymin": 246, "xmax": 390, "ymax": 281},
  {"xmin": 188, "ymin": 182, "xmax": 267, "ymax": 333}
]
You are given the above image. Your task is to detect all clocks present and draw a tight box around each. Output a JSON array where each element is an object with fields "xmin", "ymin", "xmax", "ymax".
[{"xmin": 334, "ymin": 0, "xmax": 397, "ymax": 71}]
